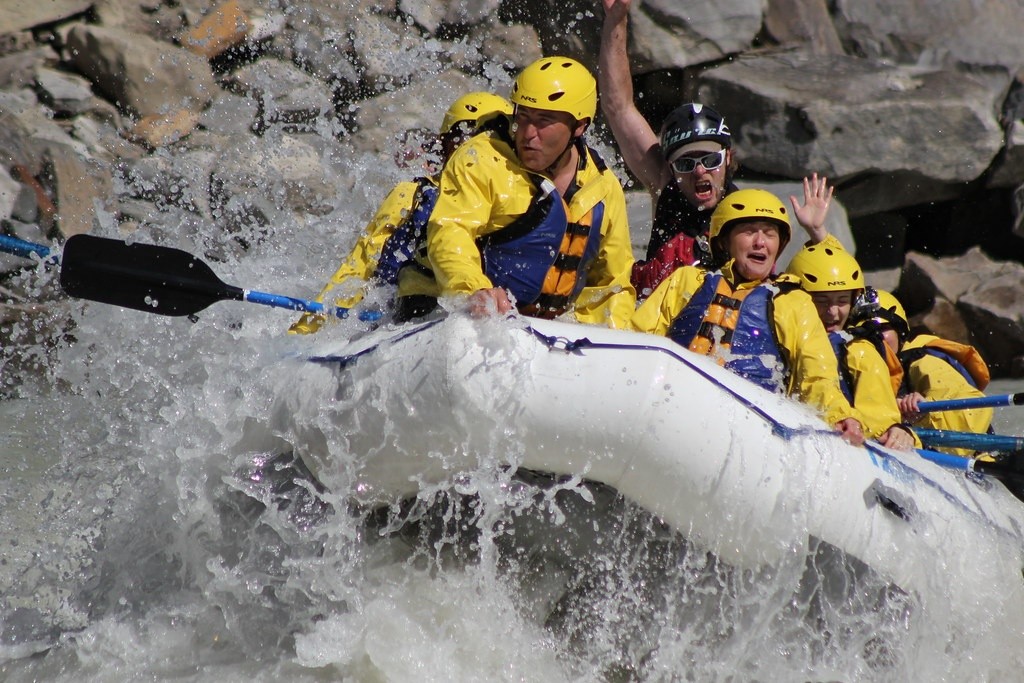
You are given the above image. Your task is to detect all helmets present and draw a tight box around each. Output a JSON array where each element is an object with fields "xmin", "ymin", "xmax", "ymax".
[
  {"xmin": 509, "ymin": 56, "xmax": 597, "ymax": 126},
  {"xmin": 708, "ymin": 189, "xmax": 792, "ymax": 260},
  {"xmin": 440, "ymin": 91, "xmax": 513, "ymax": 138},
  {"xmin": 785, "ymin": 244, "xmax": 865, "ymax": 295},
  {"xmin": 661, "ymin": 103, "xmax": 731, "ymax": 161},
  {"xmin": 846, "ymin": 287, "xmax": 910, "ymax": 334}
]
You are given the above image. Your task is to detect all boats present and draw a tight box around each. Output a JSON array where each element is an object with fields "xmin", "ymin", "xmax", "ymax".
[{"xmin": 268, "ymin": 299, "xmax": 1024, "ymax": 666}]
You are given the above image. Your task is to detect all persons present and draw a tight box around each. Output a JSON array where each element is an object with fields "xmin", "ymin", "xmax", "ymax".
[
  {"xmin": 782, "ymin": 172, "xmax": 996, "ymax": 467},
  {"xmin": 597, "ymin": 0, "xmax": 740, "ymax": 309},
  {"xmin": 784, "ymin": 240, "xmax": 923, "ymax": 452},
  {"xmin": 632, "ymin": 188, "xmax": 863, "ymax": 446},
  {"xmin": 426, "ymin": 55, "xmax": 638, "ymax": 332},
  {"xmin": 287, "ymin": 91, "xmax": 517, "ymax": 335}
]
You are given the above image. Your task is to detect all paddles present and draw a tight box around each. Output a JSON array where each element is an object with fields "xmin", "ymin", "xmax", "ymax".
[
  {"xmin": 915, "ymin": 386, "xmax": 1024, "ymax": 481},
  {"xmin": 0, "ymin": 233, "xmax": 386, "ymax": 324}
]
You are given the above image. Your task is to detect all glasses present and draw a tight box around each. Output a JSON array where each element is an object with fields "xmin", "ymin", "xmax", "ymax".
[{"xmin": 671, "ymin": 147, "xmax": 726, "ymax": 174}]
